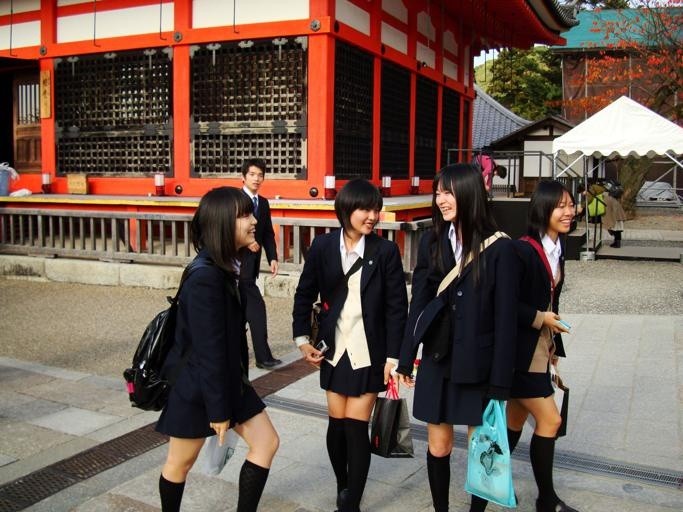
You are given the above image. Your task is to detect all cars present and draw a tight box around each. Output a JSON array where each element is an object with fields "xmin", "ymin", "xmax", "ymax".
[
  {"xmin": 635, "ymin": 180, "xmax": 679, "ymax": 204},
  {"xmin": 575, "ymin": 178, "xmax": 623, "ymax": 198}
]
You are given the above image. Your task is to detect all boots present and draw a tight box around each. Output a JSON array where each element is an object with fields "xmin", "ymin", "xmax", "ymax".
[{"xmin": 609, "ymin": 240, "xmax": 621, "ymax": 248}]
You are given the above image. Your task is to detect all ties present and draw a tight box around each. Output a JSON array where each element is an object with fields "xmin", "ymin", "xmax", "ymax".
[{"xmin": 252, "ymin": 196, "xmax": 258, "ymax": 215}]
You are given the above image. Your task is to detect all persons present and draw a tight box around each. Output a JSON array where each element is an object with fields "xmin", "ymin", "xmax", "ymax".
[
  {"xmin": 290, "ymin": 178, "xmax": 406, "ymax": 511},
  {"xmin": 468, "ymin": 179, "xmax": 576, "ymax": 511},
  {"xmin": 154, "ymin": 187, "xmax": 280, "ymax": 511},
  {"xmin": 238, "ymin": 157, "xmax": 282, "ymax": 368},
  {"xmin": 574, "ymin": 183, "xmax": 625, "ymax": 248},
  {"xmin": 472, "ymin": 155, "xmax": 506, "ymax": 198},
  {"xmin": 394, "ymin": 162, "xmax": 519, "ymax": 512}
]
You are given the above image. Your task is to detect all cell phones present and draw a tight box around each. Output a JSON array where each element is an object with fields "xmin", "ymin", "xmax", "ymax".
[{"xmin": 557, "ymin": 319, "xmax": 570, "ymax": 330}]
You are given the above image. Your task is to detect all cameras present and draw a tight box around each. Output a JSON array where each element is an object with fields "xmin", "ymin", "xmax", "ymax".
[{"xmin": 314, "ymin": 340, "xmax": 330, "ymax": 353}]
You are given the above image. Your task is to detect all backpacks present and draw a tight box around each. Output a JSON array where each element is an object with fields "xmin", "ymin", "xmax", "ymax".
[
  {"xmin": 599, "ymin": 177, "xmax": 625, "ymax": 198},
  {"xmin": 123, "ymin": 259, "xmax": 208, "ymax": 412}
]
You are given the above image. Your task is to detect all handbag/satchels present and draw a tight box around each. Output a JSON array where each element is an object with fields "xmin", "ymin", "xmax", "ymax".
[
  {"xmin": 371, "ymin": 396, "xmax": 415, "ymax": 459},
  {"xmin": 474, "ymin": 148, "xmax": 493, "ymax": 156},
  {"xmin": 309, "ymin": 303, "xmax": 328, "ymax": 348},
  {"xmin": 587, "ymin": 194, "xmax": 606, "ymax": 217},
  {"xmin": 526, "ymin": 381, "xmax": 569, "ymax": 440}
]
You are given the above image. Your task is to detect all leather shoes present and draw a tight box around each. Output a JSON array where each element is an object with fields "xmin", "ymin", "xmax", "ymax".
[
  {"xmin": 536, "ymin": 491, "xmax": 570, "ymax": 511},
  {"xmin": 256, "ymin": 359, "xmax": 281, "ymax": 369}
]
